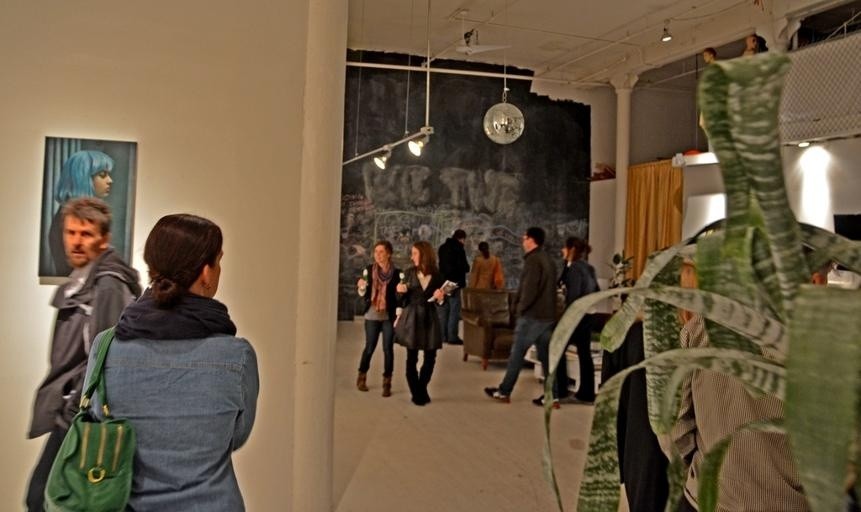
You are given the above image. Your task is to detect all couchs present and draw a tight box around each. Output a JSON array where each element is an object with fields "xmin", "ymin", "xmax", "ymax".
[{"xmin": 458, "ymin": 287, "xmax": 567, "ymax": 369}]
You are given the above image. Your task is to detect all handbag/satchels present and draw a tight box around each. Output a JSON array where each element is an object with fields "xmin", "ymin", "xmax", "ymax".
[
  {"xmin": 354, "ymin": 294, "xmax": 370, "ymax": 315},
  {"xmin": 396, "ymin": 283, "xmax": 412, "ymax": 308},
  {"xmin": 493, "ymin": 267, "xmax": 505, "ymax": 288},
  {"xmin": 43, "ymin": 405, "xmax": 135, "ymax": 512}
]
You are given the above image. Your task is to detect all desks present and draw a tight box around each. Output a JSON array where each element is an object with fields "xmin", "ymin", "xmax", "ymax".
[{"xmin": 524, "ymin": 341, "xmax": 604, "ymax": 395}]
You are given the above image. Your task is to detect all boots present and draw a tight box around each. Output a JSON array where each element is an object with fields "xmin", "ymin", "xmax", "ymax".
[
  {"xmin": 357, "ymin": 373, "xmax": 368, "ymax": 391},
  {"xmin": 406, "ymin": 367, "xmax": 430, "ymax": 405},
  {"xmin": 382, "ymin": 375, "xmax": 391, "ymax": 396}
]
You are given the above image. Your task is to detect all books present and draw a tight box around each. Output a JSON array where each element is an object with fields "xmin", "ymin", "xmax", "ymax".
[{"xmin": 426, "ymin": 280, "xmax": 458, "ymax": 303}]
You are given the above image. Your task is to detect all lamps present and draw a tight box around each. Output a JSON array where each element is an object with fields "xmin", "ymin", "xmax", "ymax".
[
  {"xmin": 343, "ymin": 1, "xmax": 434, "ymax": 170},
  {"xmin": 661, "ymin": 19, "xmax": 672, "ymax": 43}
]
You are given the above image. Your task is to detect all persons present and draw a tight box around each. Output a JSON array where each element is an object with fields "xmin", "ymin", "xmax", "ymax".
[
  {"xmin": 483, "ymin": 226, "xmax": 561, "ymax": 410},
  {"xmin": 23, "ymin": 199, "xmax": 140, "ymax": 512},
  {"xmin": 436, "ymin": 228, "xmax": 469, "ymax": 345},
  {"xmin": 354, "ymin": 240, "xmax": 402, "ymax": 398},
  {"xmin": 556, "ymin": 235, "xmax": 601, "ymax": 407},
  {"xmin": 394, "ymin": 240, "xmax": 442, "ymax": 406},
  {"xmin": 44, "ymin": 150, "xmax": 116, "ymax": 272},
  {"xmin": 78, "ymin": 214, "xmax": 260, "ymax": 512},
  {"xmin": 700, "ymin": 46, "xmax": 719, "ymax": 67},
  {"xmin": 467, "ymin": 240, "xmax": 501, "ymax": 290},
  {"xmin": 668, "ymin": 318, "xmax": 811, "ymax": 512},
  {"xmin": 736, "ymin": 35, "xmax": 759, "ymax": 58}
]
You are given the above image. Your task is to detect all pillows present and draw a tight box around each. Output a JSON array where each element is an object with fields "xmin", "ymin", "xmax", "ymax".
[
  {"xmin": 467, "ymin": 292, "xmax": 511, "ymax": 329},
  {"xmin": 509, "ymin": 292, "xmax": 520, "ymax": 324}
]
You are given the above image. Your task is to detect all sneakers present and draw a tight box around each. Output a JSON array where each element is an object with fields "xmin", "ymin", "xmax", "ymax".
[
  {"xmin": 532, "ymin": 394, "xmax": 594, "ymax": 408},
  {"xmin": 485, "ymin": 388, "xmax": 509, "ymax": 402},
  {"xmin": 448, "ymin": 336, "xmax": 463, "ymax": 345}
]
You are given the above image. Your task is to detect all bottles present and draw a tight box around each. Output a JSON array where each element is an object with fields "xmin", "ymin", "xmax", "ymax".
[
  {"xmin": 397, "ymin": 272, "xmax": 407, "ymax": 297},
  {"xmin": 359, "ymin": 268, "xmax": 369, "ymax": 291}
]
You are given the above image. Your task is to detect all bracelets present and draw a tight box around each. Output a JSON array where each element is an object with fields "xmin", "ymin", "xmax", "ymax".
[{"xmin": 436, "ymin": 298, "xmax": 443, "ymax": 303}]
